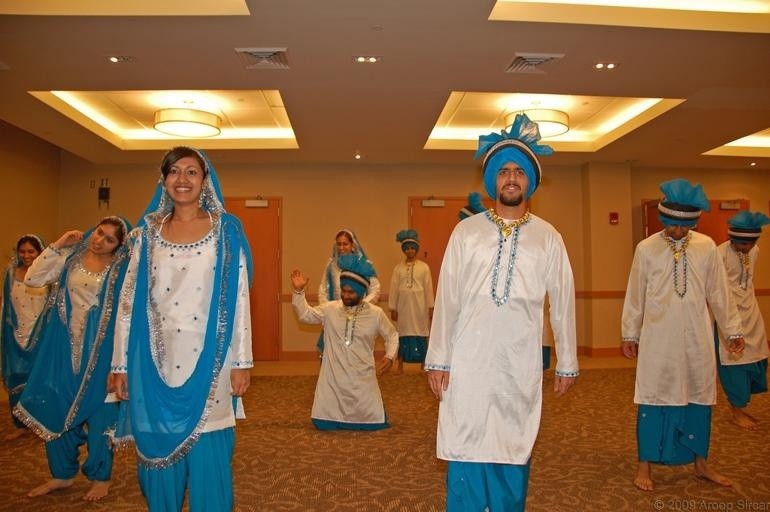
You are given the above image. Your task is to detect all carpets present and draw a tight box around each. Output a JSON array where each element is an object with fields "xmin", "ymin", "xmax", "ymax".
[{"xmin": 0, "ymin": 366, "xmax": 770, "ymax": 508}]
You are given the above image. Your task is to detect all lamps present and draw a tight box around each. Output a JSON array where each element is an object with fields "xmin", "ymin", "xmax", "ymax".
[
  {"xmin": 152, "ymin": 99, "xmax": 223, "ymax": 138},
  {"xmin": 503, "ymin": 100, "xmax": 570, "ymax": 140}
]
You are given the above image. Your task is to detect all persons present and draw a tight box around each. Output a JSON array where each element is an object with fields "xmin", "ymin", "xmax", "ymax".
[
  {"xmin": 115, "ymin": 144, "xmax": 256, "ymax": 512},
  {"xmin": 21, "ymin": 213, "xmax": 134, "ymax": 501},
  {"xmin": 714, "ymin": 207, "xmax": 770, "ymax": 432},
  {"xmin": 420, "ymin": 116, "xmax": 584, "ymax": 509},
  {"xmin": 387, "ymin": 226, "xmax": 437, "ymax": 371},
  {"xmin": 286, "ymin": 256, "xmax": 400, "ymax": 430},
  {"xmin": 316, "ymin": 226, "xmax": 383, "ymax": 367},
  {"xmin": 0, "ymin": 232, "xmax": 53, "ymax": 442},
  {"xmin": 617, "ymin": 177, "xmax": 747, "ymax": 493}
]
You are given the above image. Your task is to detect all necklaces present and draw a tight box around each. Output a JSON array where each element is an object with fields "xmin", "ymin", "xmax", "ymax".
[
  {"xmin": 737, "ymin": 247, "xmax": 753, "ymax": 292},
  {"xmin": 340, "ymin": 303, "xmax": 364, "ymax": 348},
  {"xmin": 405, "ymin": 257, "xmax": 415, "ymax": 288},
  {"xmin": 486, "ymin": 204, "xmax": 531, "ymax": 308},
  {"xmin": 662, "ymin": 230, "xmax": 695, "ymax": 299}
]
